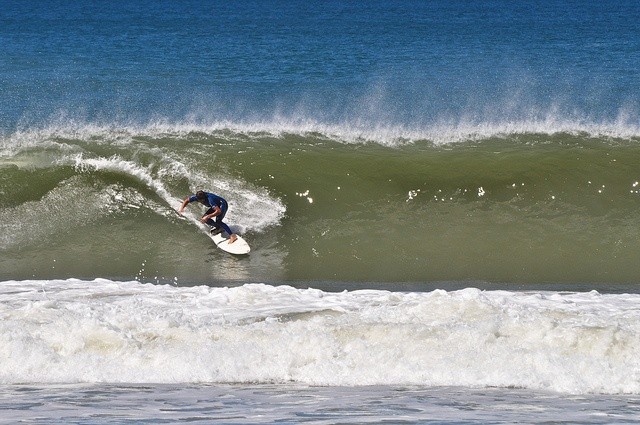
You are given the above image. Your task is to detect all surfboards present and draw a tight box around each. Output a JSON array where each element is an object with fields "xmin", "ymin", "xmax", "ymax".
[{"xmin": 205, "ymin": 227, "xmax": 251, "ymax": 255}]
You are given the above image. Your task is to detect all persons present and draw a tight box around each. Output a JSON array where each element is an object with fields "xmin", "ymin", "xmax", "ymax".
[{"xmin": 179, "ymin": 190, "xmax": 237, "ymax": 244}]
